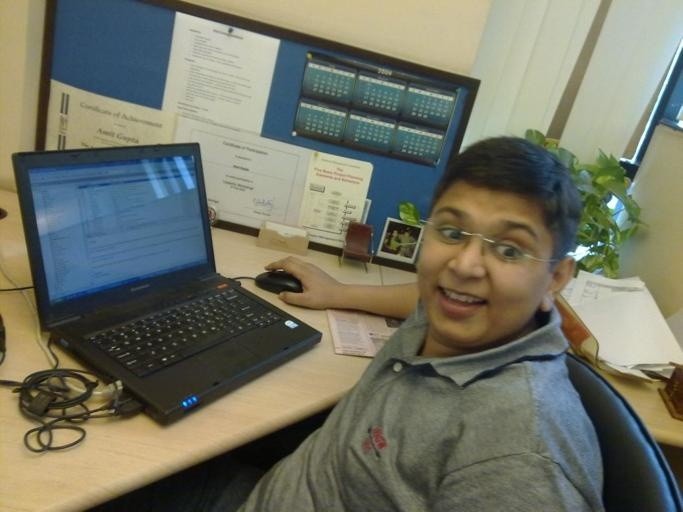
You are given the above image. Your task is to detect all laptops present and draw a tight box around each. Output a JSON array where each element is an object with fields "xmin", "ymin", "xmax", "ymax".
[{"xmin": 10, "ymin": 141, "xmax": 324, "ymax": 427}]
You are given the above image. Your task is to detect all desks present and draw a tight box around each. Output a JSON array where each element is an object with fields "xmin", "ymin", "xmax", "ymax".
[{"xmin": 0, "ymin": 186, "xmax": 682, "ymax": 512}]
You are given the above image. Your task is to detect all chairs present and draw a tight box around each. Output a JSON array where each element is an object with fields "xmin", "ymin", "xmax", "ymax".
[
  {"xmin": 566, "ymin": 350, "xmax": 682, "ymax": 512},
  {"xmin": 339, "ymin": 221, "xmax": 374, "ymax": 273}
]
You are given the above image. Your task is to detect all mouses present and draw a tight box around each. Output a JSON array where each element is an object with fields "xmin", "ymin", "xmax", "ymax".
[{"xmin": 254, "ymin": 270, "xmax": 303, "ymax": 295}]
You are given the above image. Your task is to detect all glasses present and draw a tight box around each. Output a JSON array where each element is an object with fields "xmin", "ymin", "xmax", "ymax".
[{"xmin": 422, "ymin": 219, "xmax": 558, "ymax": 264}]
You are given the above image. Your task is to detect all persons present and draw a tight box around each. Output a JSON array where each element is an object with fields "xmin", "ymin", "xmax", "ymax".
[
  {"xmin": 384, "ymin": 229, "xmax": 399, "ymax": 253},
  {"xmin": 386, "ymin": 227, "xmax": 414, "ymax": 258},
  {"xmin": 158, "ymin": 136, "xmax": 607, "ymax": 510}
]
